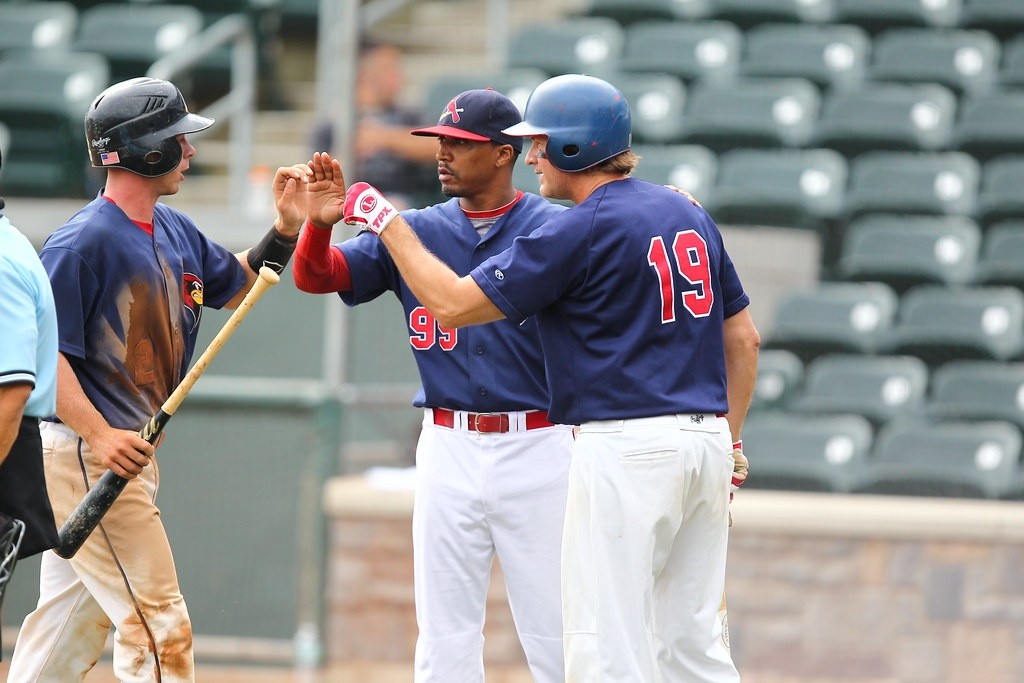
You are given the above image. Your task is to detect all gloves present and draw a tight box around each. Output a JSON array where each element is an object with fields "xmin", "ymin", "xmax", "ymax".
[
  {"xmin": 728, "ymin": 439, "xmax": 751, "ymax": 528},
  {"xmin": 342, "ymin": 180, "xmax": 400, "ymax": 237}
]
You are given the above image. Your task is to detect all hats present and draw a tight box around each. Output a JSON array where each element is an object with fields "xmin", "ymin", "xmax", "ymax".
[{"xmin": 409, "ymin": 88, "xmax": 524, "ymax": 154}]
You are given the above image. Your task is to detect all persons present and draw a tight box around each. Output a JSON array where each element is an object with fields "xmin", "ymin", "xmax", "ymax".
[
  {"xmin": 8, "ymin": 75, "xmax": 315, "ymax": 683},
  {"xmin": 291, "ymin": 86, "xmax": 702, "ymax": 683},
  {"xmin": 339, "ymin": 73, "xmax": 762, "ymax": 682},
  {"xmin": 0, "ymin": 207, "xmax": 64, "ymax": 578}
]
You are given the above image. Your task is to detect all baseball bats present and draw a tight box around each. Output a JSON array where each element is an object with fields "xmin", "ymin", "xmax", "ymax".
[{"xmin": 50, "ymin": 267, "xmax": 281, "ymax": 559}]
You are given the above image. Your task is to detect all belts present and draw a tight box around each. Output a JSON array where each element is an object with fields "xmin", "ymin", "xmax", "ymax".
[
  {"xmin": 714, "ymin": 411, "xmax": 727, "ymax": 419},
  {"xmin": 432, "ymin": 407, "xmax": 554, "ymax": 433}
]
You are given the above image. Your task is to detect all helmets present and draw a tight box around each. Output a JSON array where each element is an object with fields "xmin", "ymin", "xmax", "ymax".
[
  {"xmin": 501, "ymin": 73, "xmax": 632, "ymax": 172},
  {"xmin": 83, "ymin": 77, "xmax": 215, "ymax": 178}
]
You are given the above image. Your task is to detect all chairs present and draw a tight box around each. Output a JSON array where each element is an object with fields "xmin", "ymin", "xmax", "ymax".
[
  {"xmin": 0, "ymin": 0, "xmax": 320, "ymax": 199},
  {"xmin": 386, "ymin": 0, "xmax": 1024, "ymax": 494}
]
